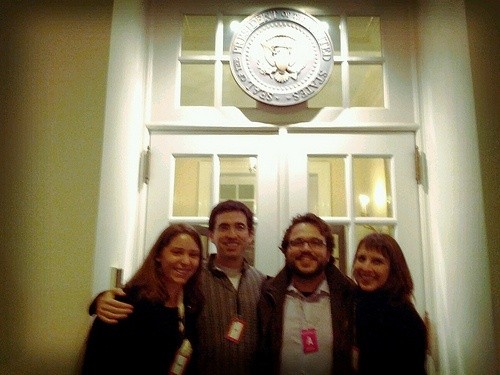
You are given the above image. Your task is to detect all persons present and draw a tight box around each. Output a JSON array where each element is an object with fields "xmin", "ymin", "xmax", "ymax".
[
  {"xmin": 88, "ymin": 198, "xmax": 275, "ymax": 375},
  {"xmin": 74, "ymin": 221, "xmax": 200, "ymax": 375},
  {"xmin": 258, "ymin": 213, "xmax": 361, "ymax": 375},
  {"xmin": 342, "ymin": 231, "xmax": 431, "ymax": 375}
]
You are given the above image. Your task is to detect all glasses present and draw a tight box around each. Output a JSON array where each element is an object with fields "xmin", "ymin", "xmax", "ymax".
[{"xmin": 287, "ymin": 237, "xmax": 328, "ymax": 248}]
